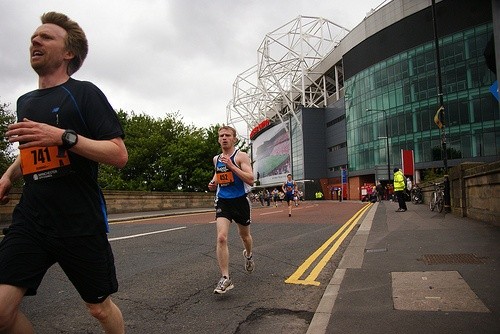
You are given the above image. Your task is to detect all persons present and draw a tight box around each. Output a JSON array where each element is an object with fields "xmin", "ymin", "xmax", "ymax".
[
  {"xmin": 393, "ymin": 167, "xmax": 408, "ymax": 212},
  {"xmin": 207, "ymin": 125, "xmax": 255, "ymax": 295},
  {"xmin": 371, "ymin": 178, "xmax": 412, "ymax": 204},
  {"xmin": 248, "ymin": 173, "xmax": 342, "ymax": 216},
  {"xmin": 0, "ymin": 11, "xmax": 123, "ymax": 334}
]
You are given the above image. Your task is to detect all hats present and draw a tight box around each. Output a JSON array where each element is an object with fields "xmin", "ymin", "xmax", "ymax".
[{"xmin": 393, "ymin": 168, "xmax": 399, "ymax": 172}]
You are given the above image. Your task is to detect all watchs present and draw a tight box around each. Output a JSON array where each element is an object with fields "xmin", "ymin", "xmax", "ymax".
[{"xmin": 61, "ymin": 129, "xmax": 78, "ymax": 149}]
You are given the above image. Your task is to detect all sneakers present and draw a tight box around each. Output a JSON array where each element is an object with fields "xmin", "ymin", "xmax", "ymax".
[
  {"xmin": 213, "ymin": 276, "xmax": 234, "ymax": 294},
  {"xmin": 243, "ymin": 249, "xmax": 254, "ymax": 273}
]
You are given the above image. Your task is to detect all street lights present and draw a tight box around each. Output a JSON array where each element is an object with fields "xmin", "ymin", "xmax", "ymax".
[
  {"xmin": 287, "ymin": 104, "xmax": 304, "ymax": 184},
  {"xmin": 366, "ymin": 108, "xmax": 390, "ymax": 184}
]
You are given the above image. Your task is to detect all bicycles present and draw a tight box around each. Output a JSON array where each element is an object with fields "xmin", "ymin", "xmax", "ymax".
[
  {"xmin": 429, "ymin": 184, "xmax": 447, "ymax": 213},
  {"xmin": 410, "ymin": 186, "xmax": 424, "ymax": 205}
]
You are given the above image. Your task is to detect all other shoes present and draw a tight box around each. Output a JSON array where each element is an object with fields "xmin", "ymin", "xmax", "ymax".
[
  {"xmin": 395, "ymin": 208, "xmax": 401, "ymax": 212},
  {"xmin": 289, "ymin": 213, "xmax": 291, "ymax": 217},
  {"xmin": 400, "ymin": 209, "xmax": 406, "ymax": 211}
]
